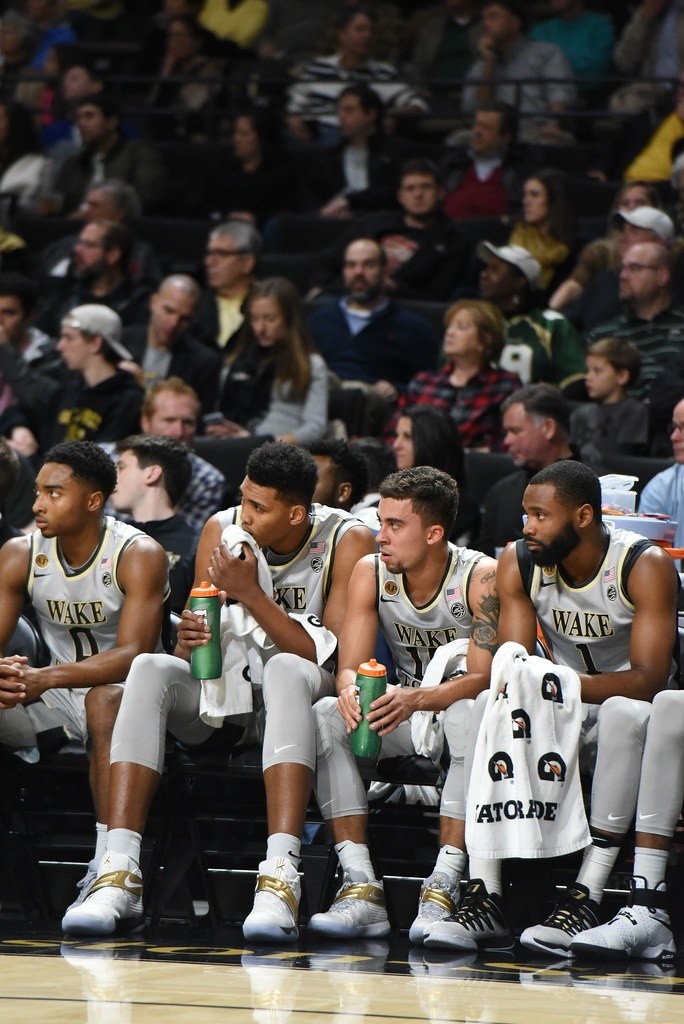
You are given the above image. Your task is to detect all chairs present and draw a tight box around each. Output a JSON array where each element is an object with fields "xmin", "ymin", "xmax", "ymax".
[
  {"xmin": 137, "ymin": 216, "xmax": 672, "ymax": 510},
  {"xmin": 8, "ymin": 749, "xmax": 446, "ymax": 943}
]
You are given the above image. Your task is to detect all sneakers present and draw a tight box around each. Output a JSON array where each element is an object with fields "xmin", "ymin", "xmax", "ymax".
[
  {"xmin": 242, "ymin": 856, "xmax": 304, "ymax": 940},
  {"xmin": 519, "ymin": 881, "xmax": 602, "ymax": 958},
  {"xmin": 569, "ymin": 876, "xmax": 676, "ymax": 962},
  {"xmin": 61, "ymin": 853, "xmax": 144, "ymax": 935},
  {"xmin": 310, "ymin": 868, "xmax": 391, "ymax": 938},
  {"xmin": 423, "ymin": 877, "xmax": 515, "ymax": 950},
  {"xmin": 408, "ymin": 874, "xmax": 460, "ymax": 942}
]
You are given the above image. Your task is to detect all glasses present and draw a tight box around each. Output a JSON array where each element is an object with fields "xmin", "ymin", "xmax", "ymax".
[{"xmin": 208, "ymin": 248, "xmax": 251, "ymax": 259}]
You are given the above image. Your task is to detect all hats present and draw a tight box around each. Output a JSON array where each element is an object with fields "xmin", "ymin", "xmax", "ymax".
[
  {"xmin": 476, "ymin": 241, "xmax": 541, "ymax": 283},
  {"xmin": 612, "ymin": 205, "xmax": 674, "ymax": 242},
  {"xmin": 61, "ymin": 303, "xmax": 133, "ymax": 362}
]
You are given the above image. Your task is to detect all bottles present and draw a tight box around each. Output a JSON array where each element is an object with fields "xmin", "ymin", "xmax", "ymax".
[
  {"xmin": 189, "ymin": 581, "xmax": 222, "ymax": 680},
  {"xmin": 348, "ymin": 657, "xmax": 388, "ymax": 757}
]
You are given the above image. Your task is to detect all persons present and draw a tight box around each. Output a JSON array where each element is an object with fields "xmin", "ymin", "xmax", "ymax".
[
  {"xmin": 312, "ymin": 468, "xmax": 498, "ymax": 940},
  {"xmin": 424, "ymin": 461, "xmax": 681, "ymax": 960},
  {"xmin": 0, "ymin": 0, "xmax": 684, "ymax": 656},
  {"xmin": 61, "ymin": 439, "xmax": 377, "ymax": 943},
  {"xmin": 570, "ymin": 690, "xmax": 684, "ymax": 963},
  {"xmin": 1, "ymin": 442, "xmax": 175, "ymax": 916}
]
